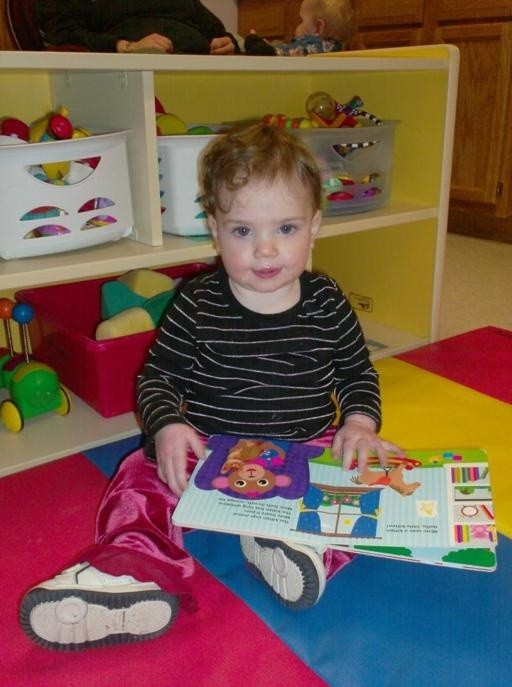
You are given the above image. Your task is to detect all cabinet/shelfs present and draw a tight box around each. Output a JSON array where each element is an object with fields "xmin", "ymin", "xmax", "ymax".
[
  {"xmin": 0, "ymin": 41, "xmax": 459, "ymax": 348},
  {"xmin": 239, "ymin": 0, "xmax": 512, "ymax": 244}
]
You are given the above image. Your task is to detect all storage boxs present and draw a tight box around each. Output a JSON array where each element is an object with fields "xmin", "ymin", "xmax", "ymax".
[
  {"xmin": 15, "ymin": 262, "xmax": 221, "ymax": 419},
  {"xmin": 153, "ymin": 123, "xmax": 232, "ymax": 237},
  {"xmin": 228, "ymin": 116, "xmax": 400, "ymax": 217},
  {"xmin": 0, "ymin": 125, "xmax": 133, "ymax": 260}
]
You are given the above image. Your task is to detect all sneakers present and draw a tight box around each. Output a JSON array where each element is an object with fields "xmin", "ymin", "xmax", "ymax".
[
  {"xmin": 240, "ymin": 532, "xmax": 325, "ymax": 610},
  {"xmin": 19, "ymin": 564, "xmax": 179, "ymax": 648}
]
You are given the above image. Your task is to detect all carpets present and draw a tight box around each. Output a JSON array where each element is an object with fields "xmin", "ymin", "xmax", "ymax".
[{"xmin": 0, "ymin": 326, "xmax": 512, "ymax": 686}]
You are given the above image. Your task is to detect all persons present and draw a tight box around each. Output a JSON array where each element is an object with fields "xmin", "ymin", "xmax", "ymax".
[
  {"xmin": 19, "ymin": 124, "xmax": 405, "ymax": 652},
  {"xmin": 245, "ymin": 0, "xmax": 357, "ymax": 56},
  {"xmin": 34, "ymin": 0, "xmax": 244, "ymax": 56}
]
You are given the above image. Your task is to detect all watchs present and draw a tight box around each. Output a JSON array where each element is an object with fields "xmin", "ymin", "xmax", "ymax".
[{"xmin": 123, "ymin": 40, "xmax": 131, "ymax": 54}]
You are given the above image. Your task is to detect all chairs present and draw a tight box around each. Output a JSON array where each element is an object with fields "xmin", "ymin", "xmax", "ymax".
[{"xmin": 6, "ymin": 1, "xmax": 52, "ymax": 52}]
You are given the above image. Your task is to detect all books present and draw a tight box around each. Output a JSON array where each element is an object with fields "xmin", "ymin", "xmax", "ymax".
[{"xmin": 171, "ymin": 434, "xmax": 496, "ymax": 573}]
